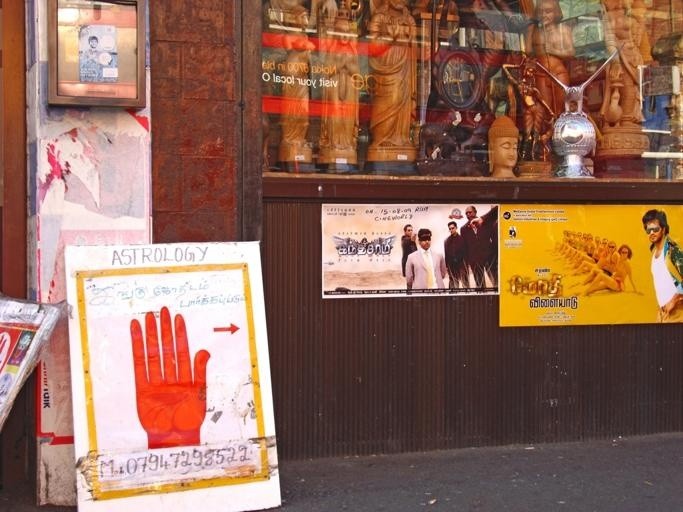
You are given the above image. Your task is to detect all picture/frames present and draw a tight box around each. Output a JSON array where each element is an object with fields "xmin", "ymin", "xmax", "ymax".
[{"xmin": 45, "ymin": 0, "xmax": 148, "ymax": 111}]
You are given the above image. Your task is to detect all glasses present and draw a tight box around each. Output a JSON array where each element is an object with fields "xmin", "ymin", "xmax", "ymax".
[
  {"xmin": 646, "ymin": 227, "xmax": 661, "ymax": 234},
  {"xmin": 561, "ymin": 233, "xmax": 630, "ymax": 255},
  {"xmin": 418, "ymin": 236, "xmax": 432, "ymax": 241}
]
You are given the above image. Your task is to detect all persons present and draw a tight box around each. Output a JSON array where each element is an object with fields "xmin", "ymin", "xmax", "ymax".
[
  {"xmin": 488, "ymin": 116, "xmax": 521, "ymax": 178},
  {"xmin": 442, "ymin": 221, "xmax": 469, "ymax": 289},
  {"xmin": 366, "ymin": 0, "xmax": 417, "ymax": 147},
  {"xmin": 316, "ymin": 19, "xmax": 360, "ymax": 149},
  {"xmin": 524, "ymin": 0, "xmax": 575, "ymax": 132},
  {"xmin": 404, "ymin": 228, "xmax": 446, "ymax": 290},
  {"xmin": 399, "ymin": 224, "xmax": 418, "ymax": 277},
  {"xmin": 545, "ymin": 230, "xmax": 643, "ymax": 298},
  {"xmin": 459, "ymin": 204, "xmax": 497, "ymax": 289},
  {"xmin": 602, "ymin": 0, "xmax": 653, "ymax": 126},
  {"xmin": 278, "ymin": 4, "xmax": 314, "ymax": 149},
  {"xmin": 86, "ymin": 35, "xmax": 100, "ymax": 60},
  {"xmin": 641, "ymin": 209, "xmax": 682, "ymax": 324}
]
